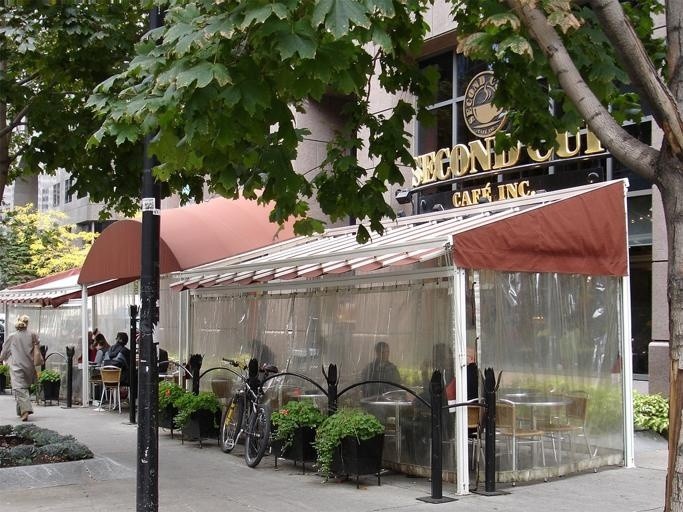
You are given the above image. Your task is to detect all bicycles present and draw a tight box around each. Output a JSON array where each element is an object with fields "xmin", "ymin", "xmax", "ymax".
[{"xmin": 216, "ymin": 356, "xmax": 280, "ymax": 468}]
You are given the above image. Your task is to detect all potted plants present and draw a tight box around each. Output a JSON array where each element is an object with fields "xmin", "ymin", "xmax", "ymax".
[
  {"xmin": 313, "ymin": 409, "xmax": 385, "ymax": 489},
  {"xmin": 171, "ymin": 391, "xmax": 224, "ymax": 449},
  {"xmin": 0, "ymin": 363, "xmax": 10, "ymax": 393},
  {"xmin": 36, "ymin": 368, "xmax": 61, "ymax": 407}
]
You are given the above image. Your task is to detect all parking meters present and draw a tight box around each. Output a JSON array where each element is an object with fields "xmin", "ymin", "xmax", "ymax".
[{"xmin": 64, "ymin": 344, "xmax": 75, "ymax": 409}]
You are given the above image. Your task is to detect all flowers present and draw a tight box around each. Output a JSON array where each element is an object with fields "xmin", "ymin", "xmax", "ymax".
[
  {"xmin": 157, "ymin": 380, "xmax": 185, "ymax": 409},
  {"xmin": 267, "ymin": 402, "xmax": 323, "ymax": 455}
]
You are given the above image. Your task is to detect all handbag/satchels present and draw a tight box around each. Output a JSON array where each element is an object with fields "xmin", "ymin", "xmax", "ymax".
[{"xmin": 31, "ymin": 333, "xmax": 44, "ymax": 367}]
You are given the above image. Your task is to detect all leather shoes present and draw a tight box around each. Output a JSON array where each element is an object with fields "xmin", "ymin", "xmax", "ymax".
[{"xmin": 21, "ymin": 412, "xmax": 28, "ymax": 421}]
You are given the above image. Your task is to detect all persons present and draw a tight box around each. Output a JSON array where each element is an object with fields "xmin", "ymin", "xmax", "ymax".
[
  {"xmin": 75, "ymin": 328, "xmax": 137, "ymax": 410},
  {"xmin": 359, "ymin": 341, "xmax": 402, "ymax": 426},
  {"xmin": 400, "ymin": 342, "xmax": 455, "ymax": 466},
  {"xmin": 1, "ymin": 313, "xmax": 41, "ymax": 421}
]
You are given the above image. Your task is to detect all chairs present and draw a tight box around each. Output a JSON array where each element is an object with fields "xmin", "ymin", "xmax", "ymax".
[
  {"xmin": 360, "ymin": 384, "xmax": 596, "ymax": 488},
  {"xmin": 86, "ymin": 362, "xmax": 123, "ymax": 414}
]
